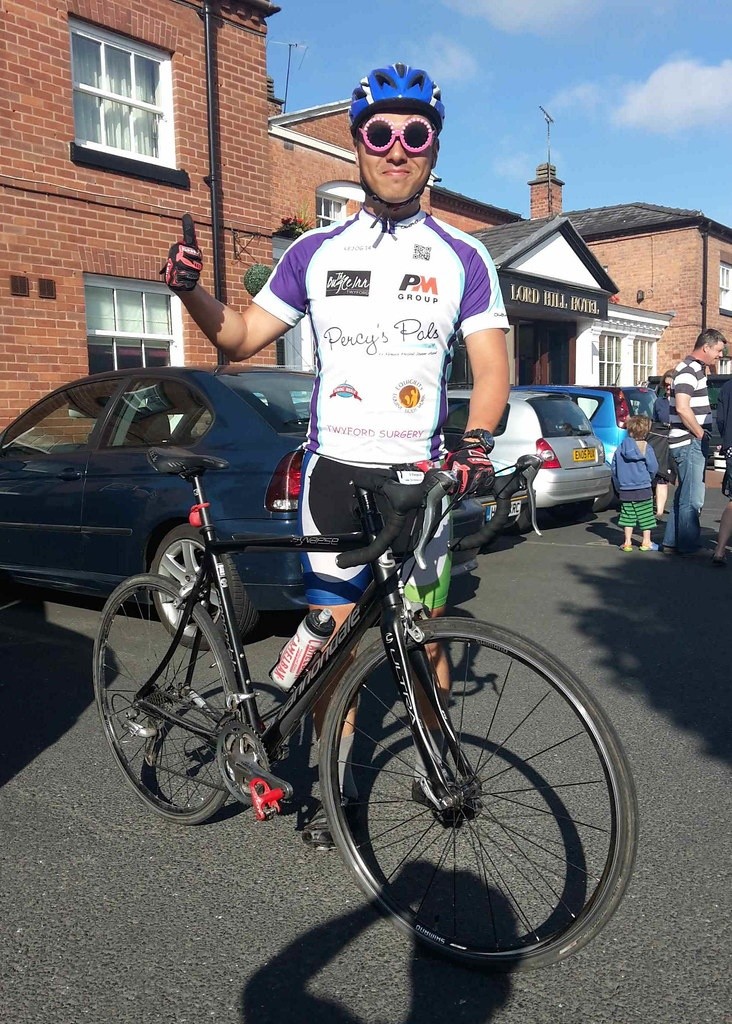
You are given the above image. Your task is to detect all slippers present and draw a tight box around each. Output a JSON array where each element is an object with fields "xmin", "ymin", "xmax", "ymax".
[
  {"xmin": 640, "ymin": 542, "xmax": 659, "ymax": 551},
  {"xmin": 620, "ymin": 544, "xmax": 633, "ymax": 551}
]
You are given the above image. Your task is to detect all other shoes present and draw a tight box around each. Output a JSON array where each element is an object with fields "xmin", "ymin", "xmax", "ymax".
[{"xmin": 711, "ymin": 551, "xmax": 728, "ymax": 567}]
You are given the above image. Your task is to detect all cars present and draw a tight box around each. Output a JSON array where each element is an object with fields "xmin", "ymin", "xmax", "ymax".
[
  {"xmin": 0, "ymin": 366, "xmax": 486, "ymax": 651},
  {"xmin": 447, "ymin": 387, "xmax": 611, "ymax": 534},
  {"xmin": 512, "ymin": 385, "xmax": 659, "ymax": 513}
]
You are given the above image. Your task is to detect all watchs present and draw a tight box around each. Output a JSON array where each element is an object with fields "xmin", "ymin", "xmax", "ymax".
[{"xmin": 461, "ymin": 429, "xmax": 495, "ymax": 454}]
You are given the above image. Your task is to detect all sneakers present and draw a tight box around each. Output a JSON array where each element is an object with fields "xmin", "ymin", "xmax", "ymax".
[
  {"xmin": 301, "ymin": 790, "xmax": 346, "ymax": 854},
  {"xmin": 682, "ymin": 547, "xmax": 714, "ymax": 557},
  {"xmin": 411, "ymin": 773, "xmax": 482, "ymax": 820},
  {"xmin": 662, "ymin": 546, "xmax": 674, "ymax": 555}
]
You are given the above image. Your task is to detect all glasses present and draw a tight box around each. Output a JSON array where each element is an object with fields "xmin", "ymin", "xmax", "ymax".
[{"xmin": 359, "ymin": 116, "xmax": 433, "ymax": 153}]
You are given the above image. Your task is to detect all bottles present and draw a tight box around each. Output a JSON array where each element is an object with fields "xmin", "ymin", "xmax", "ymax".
[{"xmin": 266, "ymin": 609, "xmax": 336, "ymax": 694}]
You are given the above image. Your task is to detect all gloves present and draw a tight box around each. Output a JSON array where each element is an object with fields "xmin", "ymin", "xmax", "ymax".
[
  {"xmin": 441, "ymin": 442, "xmax": 496, "ymax": 498},
  {"xmin": 158, "ymin": 214, "xmax": 203, "ymax": 292}
]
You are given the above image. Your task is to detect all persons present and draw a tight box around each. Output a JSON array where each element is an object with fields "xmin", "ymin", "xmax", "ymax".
[
  {"xmin": 610, "ymin": 415, "xmax": 663, "ymax": 552},
  {"xmin": 645, "ymin": 328, "xmax": 732, "ymax": 566},
  {"xmin": 164, "ymin": 61, "xmax": 512, "ymax": 852}
]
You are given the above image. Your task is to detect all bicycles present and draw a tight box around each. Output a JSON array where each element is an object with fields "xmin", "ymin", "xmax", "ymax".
[{"xmin": 92, "ymin": 446, "xmax": 639, "ymax": 972}]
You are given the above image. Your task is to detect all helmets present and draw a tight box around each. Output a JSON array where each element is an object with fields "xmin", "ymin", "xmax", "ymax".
[{"xmin": 348, "ymin": 62, "xmax": 444, "ymax": 138}]
